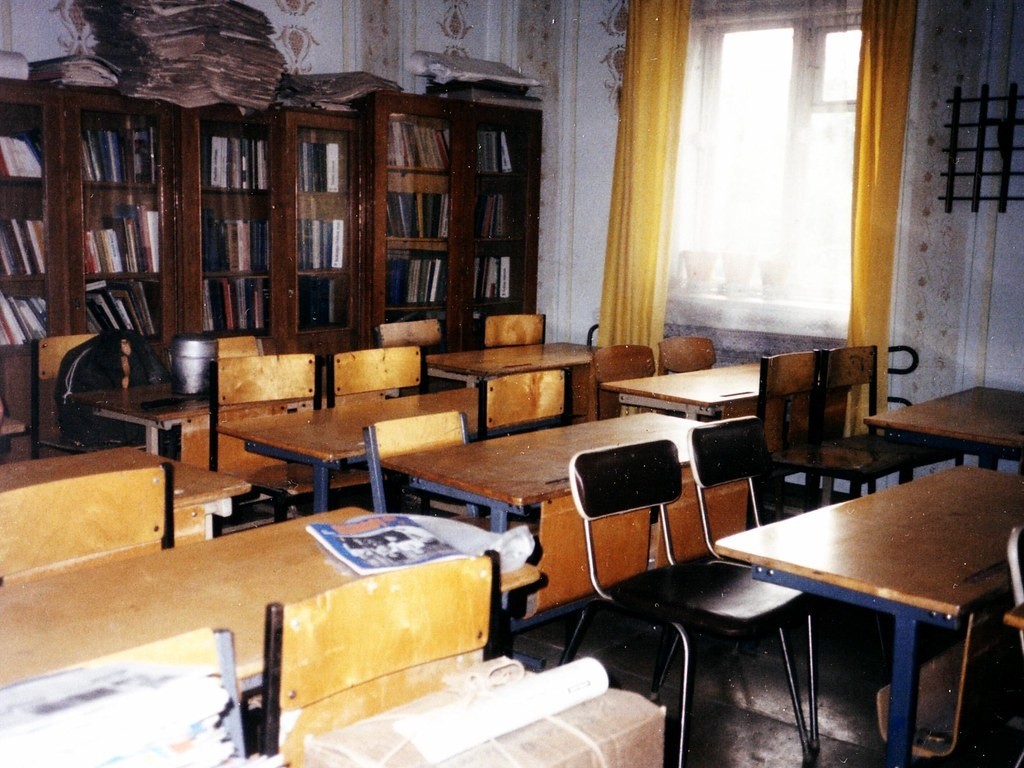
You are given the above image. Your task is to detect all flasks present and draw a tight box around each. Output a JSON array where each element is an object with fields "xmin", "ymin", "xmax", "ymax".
[{"xmin": 164, "ymin": 333, "xmax": 219, "ymax": 396}]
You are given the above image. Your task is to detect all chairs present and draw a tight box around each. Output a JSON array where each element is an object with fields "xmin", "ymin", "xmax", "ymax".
[{"xmin": 0, "ymin": 313, "xmax": 1024, "ymax": 767}]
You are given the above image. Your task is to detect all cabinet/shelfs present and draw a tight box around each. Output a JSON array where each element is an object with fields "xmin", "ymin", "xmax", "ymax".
[{"xmin": 0, "ymin": 77, "xmax": 543, "ymax": 466}]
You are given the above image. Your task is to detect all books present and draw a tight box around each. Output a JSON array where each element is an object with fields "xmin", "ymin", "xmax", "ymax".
[
  {"xmin": 305, "ymin": 515, "xmax": 470, "ymax": 576},
  {"xmin": 0, "ymin": 665, "xmax": 283, "ymax": 768},
  {"xmin": 0, "ymin": 0, "xmax": 541, "ymax": 346}
]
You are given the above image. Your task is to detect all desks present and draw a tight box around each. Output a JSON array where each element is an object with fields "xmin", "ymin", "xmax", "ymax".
[
  {"xmin": 74, "ymin": 384, "xmax": 387, "ymax": 455},
  {"xmin": 381, "ymin": 412, "xmax": 750, "ymax": 628},
  {"xmin": 599, "ymin": 365, "xmax": 759, "ymax": 420},
  {"xmin": 712, "ymin": 466, "xmax": 1024, "ymax": 768},
  {"xmin": 1, "ymin": 506, "xmax": 541, "ymax": 699},
  {"xmin": 0, "ymin": 446, "xmax": 254, "ymax": 545},
  {"xmin": 865, "ymin": 387, "xmax": 1024, "ymax": 486},
  {"xmin": 215, "ymin": 387, "xmax": 478, "ymax": 513},
  {"xmin": 424, "ymin": 341, "xmax": 603, "ymax": 387}
]
